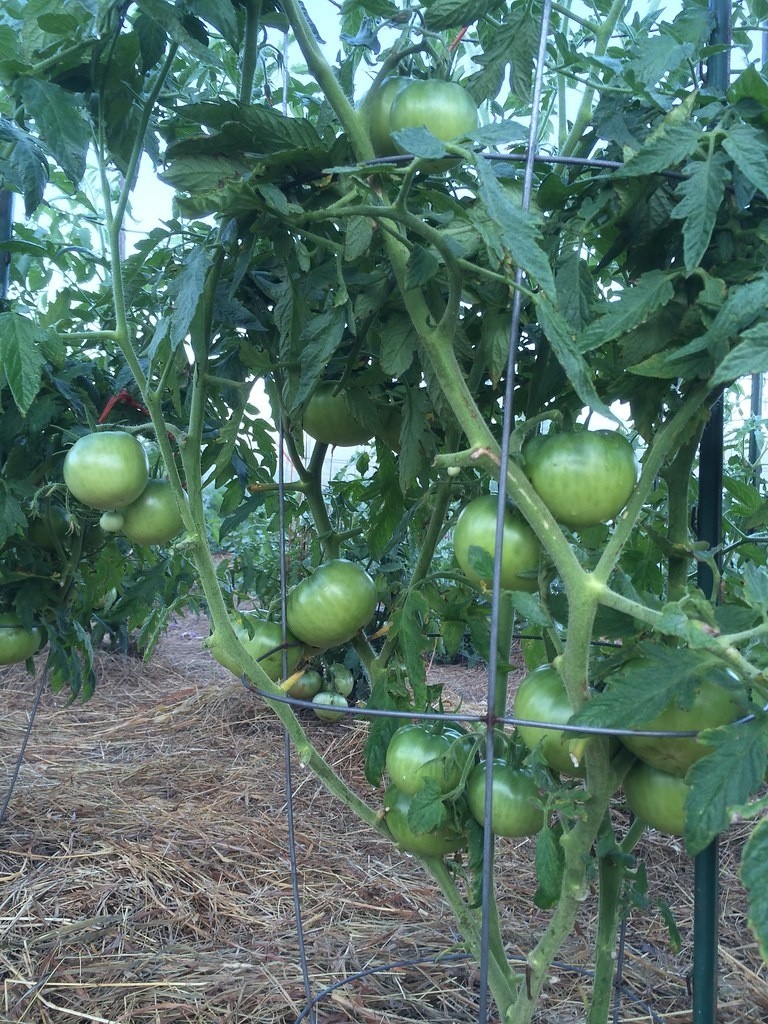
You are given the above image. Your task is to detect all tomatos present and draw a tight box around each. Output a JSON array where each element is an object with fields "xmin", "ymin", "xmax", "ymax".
[{"xmin": 0, "ymin": 76, "xmax": 744, "ymax": 858}]
